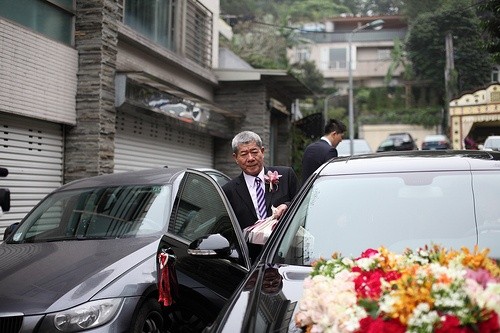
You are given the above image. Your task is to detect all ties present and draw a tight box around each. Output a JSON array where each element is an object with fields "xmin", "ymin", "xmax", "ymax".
[{"xmin": 255, "ymin": 177, "xmax": 267, "ymax": 220}]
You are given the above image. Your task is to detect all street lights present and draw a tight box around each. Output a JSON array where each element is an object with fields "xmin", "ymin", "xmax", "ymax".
[{"xmin": 348, "ymin": 19, "xmax": 385, "ymax": 156}]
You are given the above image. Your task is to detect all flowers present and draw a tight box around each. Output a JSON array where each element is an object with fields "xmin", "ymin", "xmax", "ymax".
[
  {"xmin": 263, "ymin": 170, "xmax": 283, "ymax": 193},
  {"xmin": 293, "ymin": 239, "xmax": 500, "ymax": 333}
]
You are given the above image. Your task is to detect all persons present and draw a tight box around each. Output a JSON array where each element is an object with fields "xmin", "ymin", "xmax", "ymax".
[
  {"xmin": 301, "ymin": 118, "xmax": 346, "ymax": 185},
  {"xmin": 222, "ymin": 130, "xmax": 299, "ymax": 261}
]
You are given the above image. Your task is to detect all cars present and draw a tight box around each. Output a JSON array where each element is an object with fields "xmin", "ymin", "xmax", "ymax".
[
  {"xmin": 376, "ymin": 132, "xmax": 453, "ymax": 153},
  {"xmin": 336, "ymin": 139, "xmax": 373, "ymax": 156},
  {"xmin": 156, "ymin": 150, "xmax": 500, "ymax": 333},
  {"xmin": 0, "ymin": 167, "xmax": 233, "ymax": 333},
  {"xmin": 478, "ymin": 136, "xmax": 500, "ymax": 161}
]
